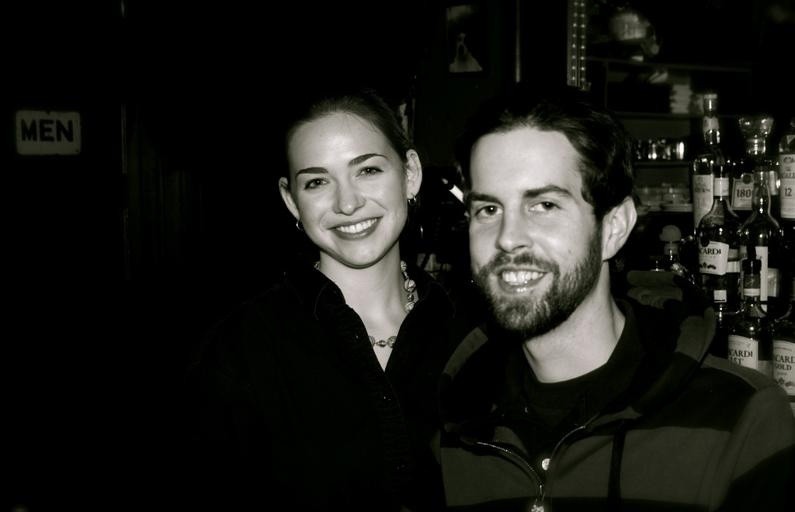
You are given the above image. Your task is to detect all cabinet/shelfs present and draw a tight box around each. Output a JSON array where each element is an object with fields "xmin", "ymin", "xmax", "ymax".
[{"xmin": 565, "ymin": 4, "xmax": 795, "ymax": 332}]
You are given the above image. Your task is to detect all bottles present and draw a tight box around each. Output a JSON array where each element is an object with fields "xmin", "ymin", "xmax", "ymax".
[{"xmin": 694, "ymin": 95, "xmax": 795, "ymax": 403}]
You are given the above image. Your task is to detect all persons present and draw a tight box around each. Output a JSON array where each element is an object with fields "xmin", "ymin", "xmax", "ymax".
[{"xmin": 274, "ymin": 81, "xmax": 793, "ymax": 511}]
